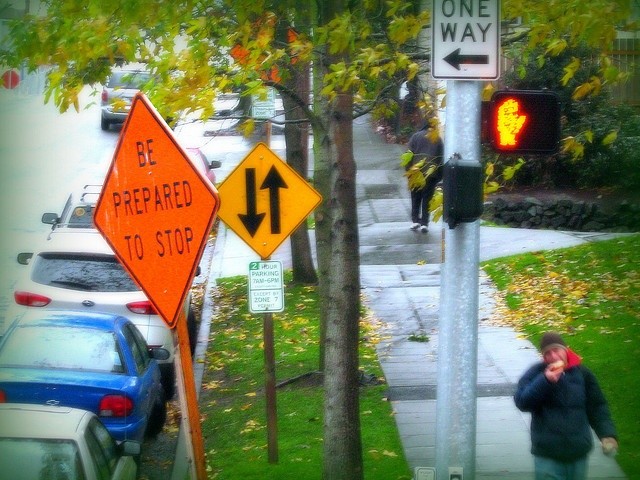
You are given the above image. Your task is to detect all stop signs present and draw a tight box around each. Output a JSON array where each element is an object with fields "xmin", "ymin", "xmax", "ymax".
[{"xmin": 92, "ymin": 91, "xmax": 222, "ymax": 331}]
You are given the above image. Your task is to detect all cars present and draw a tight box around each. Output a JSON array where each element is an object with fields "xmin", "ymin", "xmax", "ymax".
[
  {"xmin": 183, "ymin": 146, "xmax": 221, "ymax": 186},
  {"xmin": 0, "ymin": 403, "xmax": 142, "ymax": 480},
  {"xmin": 0, "ymin": 307, "xmax": 170, "ymax": 441}
]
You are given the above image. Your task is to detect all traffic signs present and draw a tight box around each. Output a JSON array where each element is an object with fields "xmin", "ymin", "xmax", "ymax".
[{"xmin": 431, "ymin": 0, "xmax": 501, "ymax": 82}]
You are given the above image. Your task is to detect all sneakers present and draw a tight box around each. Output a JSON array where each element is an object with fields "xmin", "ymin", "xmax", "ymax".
[
  {"xmin": 421, "ymin": 225, "xmax": 429, "ymax": 233},
  {"xmin": 409, "ymin": 222, "xmax": 420, "ymax": 231}
]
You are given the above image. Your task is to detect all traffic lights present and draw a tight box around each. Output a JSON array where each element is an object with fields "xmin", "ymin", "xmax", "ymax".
[
  {"xmin": 442, "ymin": 152, "xmax": 484, "ymax": 230},
  {"xmin": 490, "ymin": 89, "xmax": 562, "ymax": 156}
]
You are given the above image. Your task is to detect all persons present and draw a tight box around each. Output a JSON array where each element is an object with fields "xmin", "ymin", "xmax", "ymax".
[
  {"xmin": 407, "ymin": 113, "xmax": 444, "ymax": 233},
  {"xmin": 515, "ymin": 332, "xmax": 619, "ymax": 479}
]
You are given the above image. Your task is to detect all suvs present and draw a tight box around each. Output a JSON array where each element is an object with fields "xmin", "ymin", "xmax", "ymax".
[
  {"xmin": 40, "ymin": 182, "xmax": 103, "ymax": 229},
  {"xmin": 101, "ymin": 65, "xmax": 162, "ymax": 130},
  {"xmin": 14, "ymin": 230, "xmax": 201, "ymax": 401}
]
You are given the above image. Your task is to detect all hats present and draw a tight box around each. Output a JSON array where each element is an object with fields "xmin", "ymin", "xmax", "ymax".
[{"xmin": 540, "ymin": 332, "xmax": 568, "ymax": 357}]
